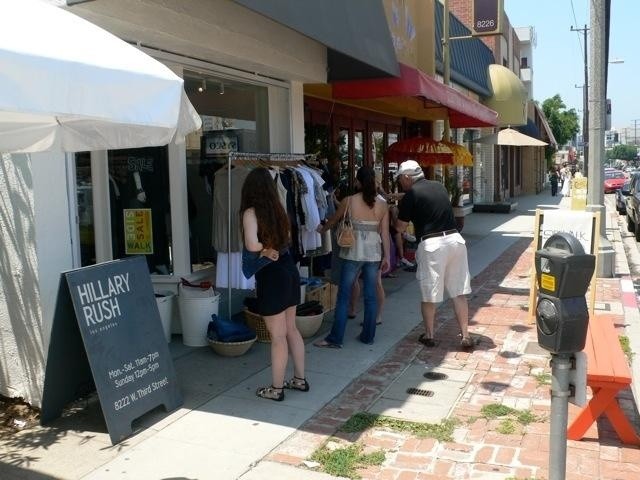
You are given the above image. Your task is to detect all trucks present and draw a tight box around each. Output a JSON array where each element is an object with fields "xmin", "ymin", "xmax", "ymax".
[{"xmin": 388, "ymin": 162, "xmax": 398, "ymax": 175}]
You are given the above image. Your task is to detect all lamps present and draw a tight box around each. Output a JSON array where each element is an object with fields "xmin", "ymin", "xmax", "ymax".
[{"xmin": 197, "ymin": 76, "xmax": 226, "ymax": 95}]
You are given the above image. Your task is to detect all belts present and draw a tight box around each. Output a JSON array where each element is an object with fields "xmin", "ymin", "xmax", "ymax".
[{"xmin": 419, "ymin": 228, "xmax": 460, "ymax": 242}]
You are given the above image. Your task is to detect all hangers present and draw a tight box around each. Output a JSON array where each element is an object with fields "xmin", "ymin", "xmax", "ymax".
[{"xmin": 219, "ymin": 149, "xmax": 312, "ymax": 169}]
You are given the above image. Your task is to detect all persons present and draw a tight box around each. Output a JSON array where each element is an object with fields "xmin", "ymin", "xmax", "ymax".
[
  {"xmin": 389, "ymin": 160, "xmax": 481, "ymax": 347},
  {"xmin": 239, "ymin": 166, "xmax": 310, "ymax": 401},
  {"xmin": 549, "ymin": 162, "xmax": 572, "ymax": 198},
  {"xmin": 313, "ymin": 165, "xmax": 416, "ymax": 348}
]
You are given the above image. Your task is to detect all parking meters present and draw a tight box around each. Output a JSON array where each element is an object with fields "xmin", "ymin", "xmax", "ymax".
[{"xmin": 532, "ymin": 230, "xmax": 598, "ymax": 480}]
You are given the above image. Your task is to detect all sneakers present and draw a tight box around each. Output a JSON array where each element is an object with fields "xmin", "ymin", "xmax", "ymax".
[
  {"xmin": 395, "ymin": 257, "xmax": 417, "ymax": 270},
  {"xmin": 461, "ymin": 335, "xmax": 473, "ymax": 347},
  {"xmin": 347, "ymin": 314, "xmax": 356, "ymax": 319},
  {"xmin": 401, "ymin": 232, "xmax": 417, "ymax": 243},
  {"xmin": 418, "ymin": 333, "xmax": 436, "ymax": 348},
  {"xmin": 359, "ymin": 321, "xmax": 383, "ymax": 326}
]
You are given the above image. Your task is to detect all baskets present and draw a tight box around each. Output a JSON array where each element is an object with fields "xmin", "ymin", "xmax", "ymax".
[
  {"xmin": 240, "ymin": 306, "xmax": 273, "ymax": 344},
  {"xmin": 205, "ymin": 334, "xmax": 260, "ymax": 358}
]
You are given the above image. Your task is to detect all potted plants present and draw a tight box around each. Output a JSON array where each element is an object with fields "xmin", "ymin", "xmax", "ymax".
[{"xmin": 433, "ymin": 170, "xmax": 488, "ymax": 218}]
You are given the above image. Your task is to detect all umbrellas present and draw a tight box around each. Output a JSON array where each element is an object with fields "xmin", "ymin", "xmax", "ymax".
[
  {"xmin": 385, "ymin": 137, "xmax": 474, "ymax": 169},
  {"xmin": 470, "ymin": 125, "xmax": 549, "ymax": 202}
]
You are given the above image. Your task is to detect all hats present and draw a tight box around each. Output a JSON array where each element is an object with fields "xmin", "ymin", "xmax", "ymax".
[{"xmin": 395, "ymin": 159, "xmax": 424, "ymax": 176}]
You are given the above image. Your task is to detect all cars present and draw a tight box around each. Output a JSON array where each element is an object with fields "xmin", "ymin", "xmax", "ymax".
[
  {"xmin": 624, "ymin": 171, "xmax": 640, "ymax": 244},
  {"xmin": 604, "ymin": 171, "xmax": 630, "ymax": 194},
  {"xmin": 615, "ymin": 179, "xmax": 632, "ymax": 216},
  {"xmin": 604, "ymin": 165, "xmax": 640, "ymax": 179}
]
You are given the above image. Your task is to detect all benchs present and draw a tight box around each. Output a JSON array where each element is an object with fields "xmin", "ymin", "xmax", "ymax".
[{"xmin": 566, "ymin": 314, "xmax": 640, "ymax": 449}]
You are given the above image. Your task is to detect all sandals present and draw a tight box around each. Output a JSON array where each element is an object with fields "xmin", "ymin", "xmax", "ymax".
[
  {"xmin": 312, "ymin": 337, "xmax": 343, "ymax": 349},
  {"xmin": 256, "ymin": 384, "xmax": 285, "ymax": 402},
  {"xmin": 283, "ymin": 374, "xmax": 310, "ymax": 392},
  {"xmin": 355, "ymin": 335, "xmax": 375, "ymax": 345}
]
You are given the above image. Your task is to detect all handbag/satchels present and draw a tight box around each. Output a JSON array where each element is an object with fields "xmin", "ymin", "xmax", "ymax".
[{"xmin": 336, "ymin": 195, "xmax": 357, "ymax": 249}]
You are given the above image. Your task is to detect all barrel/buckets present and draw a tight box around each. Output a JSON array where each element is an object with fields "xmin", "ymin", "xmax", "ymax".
[
  {"xmin": 301, "ymin": 278, "xmax": 309, "ymax": 304},
  {"xmin": 153, "ymin": 288, "xmax": 175, "ymax": 344},
  {"xmin": 176, "ymin": 290, "xmax": 222, "ymax": 348}
]
